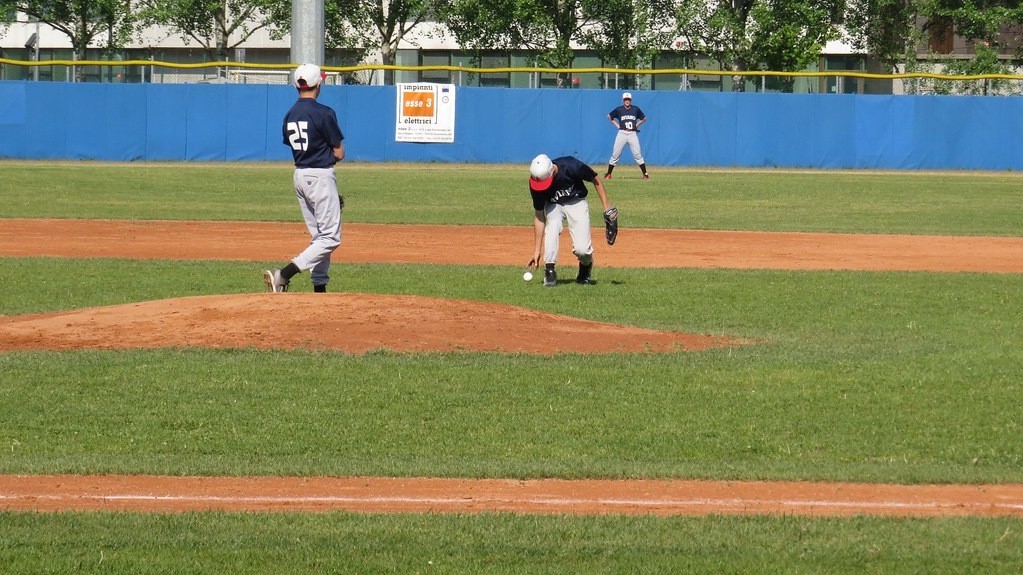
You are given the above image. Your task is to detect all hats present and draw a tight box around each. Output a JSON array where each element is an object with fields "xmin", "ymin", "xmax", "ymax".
[
  {"xmin": 623, "ymin": 93, "xmax": 632, "ymax": 100},
  {"xmin": 530, "ymin": 154, "xmax": 554, "ymax": 191},
  {"xmin": 294, "ymin": 63, "xmax": 327, "ymax": 88}
]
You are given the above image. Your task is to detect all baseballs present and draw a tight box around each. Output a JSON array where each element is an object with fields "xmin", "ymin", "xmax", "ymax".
[{"xmin": 523, "ymin": 271, "xmax": 533, "ymax": 281}]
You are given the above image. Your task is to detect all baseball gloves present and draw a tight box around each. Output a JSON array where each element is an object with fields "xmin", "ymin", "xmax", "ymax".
[{"xmin": 603, "ymin": 207, "xmax": 619, "ymax": 245}]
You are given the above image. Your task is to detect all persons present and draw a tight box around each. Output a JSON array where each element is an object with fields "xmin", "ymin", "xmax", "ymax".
[
  {"xmin": 263, "ymin": 64, "xmax": 344, "ymax": 293},
  {"xmin": 526, "ymin": 154, "xmax": 618, "ymax": 286},
  {"xmin": 604, "ymin": 92, "xmax": 650, "ymax": 179}
]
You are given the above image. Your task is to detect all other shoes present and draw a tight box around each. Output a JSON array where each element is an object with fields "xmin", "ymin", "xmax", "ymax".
[
  {"xmin": 643, "ymin": 173, "xmax": 649, "ymax": 180},
  {"xmin": 604, "ymin": 174, "xmax": 611, "ymax": 180}
]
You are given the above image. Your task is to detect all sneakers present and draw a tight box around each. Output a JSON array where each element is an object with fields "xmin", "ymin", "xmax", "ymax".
[
  {"xmin": 264, "ymin": 268, "xmax": 291, "ymax": 292},
  {"xmin": 544, "ymin": 263, "xmax": 557, "ymax": 287},
  {"xmin": 576, "ymin": 271, "xmax": 597, "ymax": 285}
]
